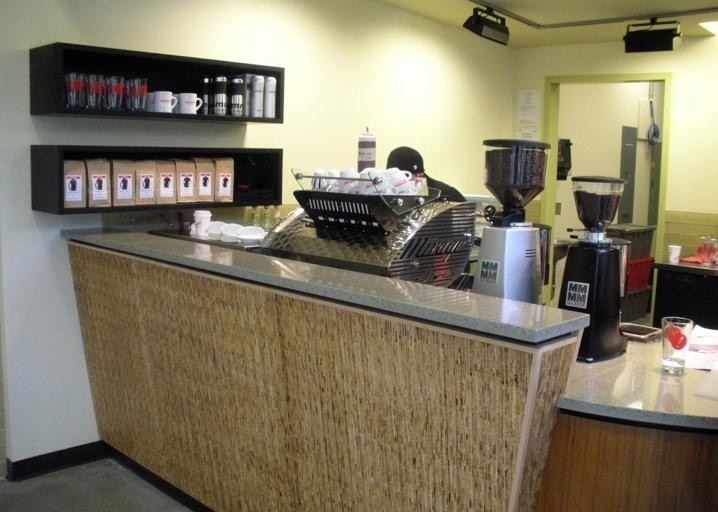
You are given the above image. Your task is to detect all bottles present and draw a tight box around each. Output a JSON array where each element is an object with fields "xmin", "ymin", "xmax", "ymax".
[
  {"xmin": 199, "ymin": 73, "xmax": 277, "ymax": 118},
  {"xmin": 357, "ymin": 124, "xmax": 376, "ymax": 173}
]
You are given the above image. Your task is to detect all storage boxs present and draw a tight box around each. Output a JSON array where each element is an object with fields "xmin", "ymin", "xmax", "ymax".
[{"xmin": 605, "ymin": 224, "xmax": 655, "ymax": 321}]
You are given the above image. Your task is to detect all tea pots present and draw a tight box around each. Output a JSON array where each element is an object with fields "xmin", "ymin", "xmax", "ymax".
[{"xmin": 67, "ymin": 175, "xmax": 229, "ymax": 192}]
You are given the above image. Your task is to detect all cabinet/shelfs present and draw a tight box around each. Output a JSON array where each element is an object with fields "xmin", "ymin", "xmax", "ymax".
[{"xmin": 651, "ymin": 270, "xmax": 717, "ymax": 330}]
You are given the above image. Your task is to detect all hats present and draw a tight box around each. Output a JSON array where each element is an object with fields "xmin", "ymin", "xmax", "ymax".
[{"xmin": 387, "ymin": 147, "xmax": 422, "ymax": 173}]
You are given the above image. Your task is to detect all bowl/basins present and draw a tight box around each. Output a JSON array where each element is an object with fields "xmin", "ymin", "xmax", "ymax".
[{"xmin": 187, "ymin": 220, "xmax": 270, "ymax": 243}]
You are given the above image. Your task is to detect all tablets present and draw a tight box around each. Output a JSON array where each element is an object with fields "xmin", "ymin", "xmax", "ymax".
[{"xmin": 620, "ymin": 322, "xmax": 662, "ymax": 341}]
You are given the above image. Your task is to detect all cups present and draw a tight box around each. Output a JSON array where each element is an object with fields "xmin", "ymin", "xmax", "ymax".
[
  {"xmin": 240, "ymin": 205, "xmax": 277, "ymax": 229},
  {"xmin": 667, "ymin": 244, "xmax": 681, "ymax": 265},
  {"xmin": 311, "ymin": 166, "xmax": 428, "ymax": 197},
  {"xmin": 660, "ymin": 316, "xmax": 693, "ymax": 376},
  {"xmin": 144, "ymin": 89, "xmax": 202, "ymax": 116},
  {"xmin": 193, "ymin": 209, "xmax": 212, "ymax": 242},
  {"xmin": 696, "ymin": 233, "xmax": 717, "ymax": 266},
  {"xmin": 60, "ymin": 72, "xmax": 147, "ymax": 110}
]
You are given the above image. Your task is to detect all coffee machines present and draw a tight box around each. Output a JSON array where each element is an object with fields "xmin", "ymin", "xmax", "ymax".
[
  {"xmin": 554, "ymin": 175, "xmax": 632, "ymax": 362},
  {"xmin": 468, "ymin": 137, "xmax": 552, "ymax": 303}
]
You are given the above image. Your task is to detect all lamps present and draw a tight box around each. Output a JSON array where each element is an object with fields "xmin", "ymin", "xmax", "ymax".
[
  {"xmin": 460, "ymin": 4, "xmax": 509, "ymax": 48},
  {"xmin": 622, "ymin": 17, "xmax": 682, "ymax": 52}
]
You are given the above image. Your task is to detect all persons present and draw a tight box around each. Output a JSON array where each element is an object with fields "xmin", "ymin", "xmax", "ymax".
[{"xmin": 385, "ymin": 145, "xmax": 466, "ymax": 203}]
